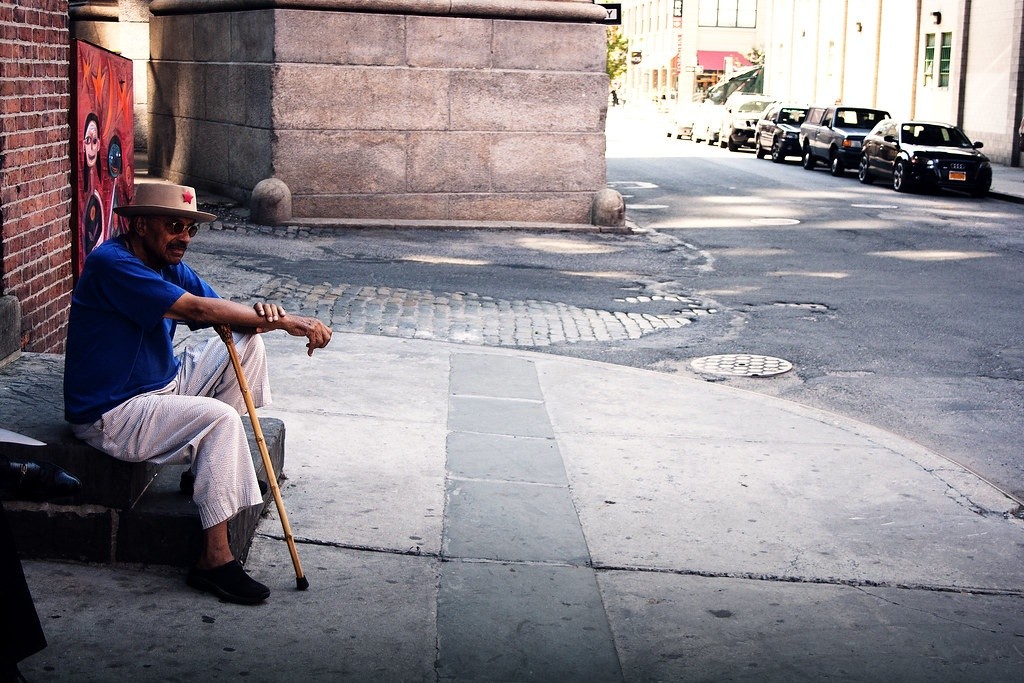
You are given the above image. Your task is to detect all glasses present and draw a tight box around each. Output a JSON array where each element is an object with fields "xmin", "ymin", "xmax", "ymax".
[{"xmin": 140, "ymin": 215, "xmax": 198, "ymax": 238}]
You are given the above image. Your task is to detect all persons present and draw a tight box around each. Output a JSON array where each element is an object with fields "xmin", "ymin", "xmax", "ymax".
[{"xmin": 63, "ymin": 178, "xmax": 334, "ymax": 607}]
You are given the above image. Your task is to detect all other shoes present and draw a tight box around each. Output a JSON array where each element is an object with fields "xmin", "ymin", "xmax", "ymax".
[
  {"xmin": 180, "ymin": 472, "xmax": 268, "ymax": 497},
  {"xmin": 186, "ymin": 563, "xmax": 271, "ymax": 605}
]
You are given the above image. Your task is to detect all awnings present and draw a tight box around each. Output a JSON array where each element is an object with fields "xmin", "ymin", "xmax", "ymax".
[
  {"xmin": 695, "ymin": 49, "xmax": 755, "ymax": 72},
  {"xmin": 726, "ymin": 64, "xmax": 762, "ymax": 81}
]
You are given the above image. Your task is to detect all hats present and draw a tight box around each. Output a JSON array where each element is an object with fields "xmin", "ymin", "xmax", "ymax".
[{"xmin": 113, "ymin": 183, "xmax": 217, "ymax": 222}]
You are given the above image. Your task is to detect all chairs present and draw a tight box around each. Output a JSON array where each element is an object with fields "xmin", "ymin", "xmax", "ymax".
[{"xmin": 780, "ymin": 112, "xmax": 939, "ymax": 144}]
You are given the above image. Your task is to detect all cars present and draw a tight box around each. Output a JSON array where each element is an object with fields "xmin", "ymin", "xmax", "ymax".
[
  {"xmin": 667, "ymin": 95, "xmax": 777, "ymax": 152},
  {"xmin": 858, "ymin": 118, "xmax": 993, "ymax": 200},
  {"xmin": 798, "ymin": 106, "xmax": 891, "ymax": 178},
  {"xmin": 755, "ymin": 104, "xmax": 808, "ymax": 163}
]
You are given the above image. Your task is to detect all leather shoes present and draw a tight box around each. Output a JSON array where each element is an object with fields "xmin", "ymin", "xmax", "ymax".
[
  {"xmin": 0, "ymin": 450, "xmax": 82, "ymax": 507},
  {"xmin": 0, "ymin": 671, "xmax": 27, "ymax": 683}
]
